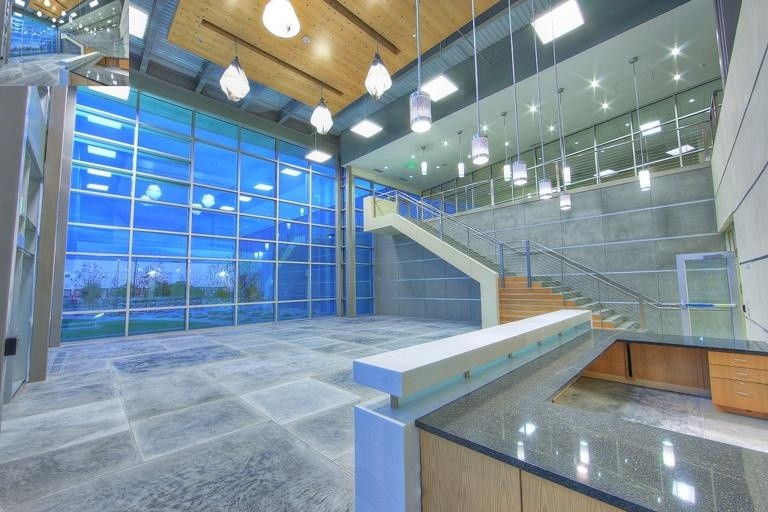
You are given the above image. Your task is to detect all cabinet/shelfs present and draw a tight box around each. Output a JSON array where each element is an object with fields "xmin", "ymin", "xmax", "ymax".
[
  {"xmin": 708, "ymin": 351, "xmax": 768, "ymax": 420},
  {"xmin": 119, "ymin": 59, "xmax": 129, "ymax": 70},
  {"xmin": 0, "ymin": 86, "xmax": 51, "ymax": 403},
  {"xmin": 0, "ymin": 0, "xmax": 13, "ymax": 64}
]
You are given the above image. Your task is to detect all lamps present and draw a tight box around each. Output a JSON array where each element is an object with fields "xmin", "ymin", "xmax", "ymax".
[
  {"xmin": 262, "ymin": 0, "xmax": 301, "ymax": 40},
  {"xmin": 408, "ymin": 89, "xmax": 433, "ymax": 135},
  {"xmin": 308, "ymin": 102, "xmax": 335, "ymax": 135},
  {"xmin": 220, "ymin": 118, "xmax": 385, "ymax": 211},
  {"xmin": 417, "ymin": 2, "xmax": 652, "ymax": 211},
  {"xmin": 364, "ymin": 59, "xmax": 394, "ymax": 100},
  {"xmin": 220, "ymin": 60, "xmax": 251, "ymax": 104}
]
